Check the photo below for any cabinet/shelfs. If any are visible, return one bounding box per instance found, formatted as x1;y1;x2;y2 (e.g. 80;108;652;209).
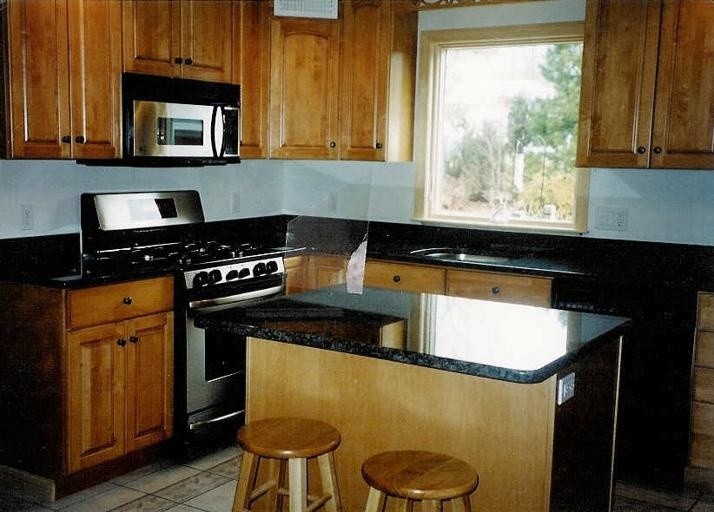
574;1;714;169
283;254;553;309
124;0;233;80
233;0;270;160
1;253;177;479
689;288;713;475
270;1;418;165
2;1;124;165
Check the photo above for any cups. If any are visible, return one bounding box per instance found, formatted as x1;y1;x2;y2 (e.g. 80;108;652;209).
543;205;556;221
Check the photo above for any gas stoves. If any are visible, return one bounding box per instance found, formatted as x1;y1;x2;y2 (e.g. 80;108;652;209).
84;239;285;290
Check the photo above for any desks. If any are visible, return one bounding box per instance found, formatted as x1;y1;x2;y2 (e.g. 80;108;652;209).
189;280;633;512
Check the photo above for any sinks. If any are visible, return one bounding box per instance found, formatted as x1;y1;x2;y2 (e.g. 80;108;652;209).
414;247;526;266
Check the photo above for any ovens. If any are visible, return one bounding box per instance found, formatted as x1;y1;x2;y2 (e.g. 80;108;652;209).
175;284;287;431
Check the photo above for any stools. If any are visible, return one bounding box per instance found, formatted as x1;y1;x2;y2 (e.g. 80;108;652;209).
359;446;482;511
226;412;350;511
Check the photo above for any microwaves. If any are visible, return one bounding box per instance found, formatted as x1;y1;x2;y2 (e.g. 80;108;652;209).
122;72;240;166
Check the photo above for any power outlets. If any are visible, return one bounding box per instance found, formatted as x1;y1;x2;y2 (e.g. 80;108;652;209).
556;371;576;406
